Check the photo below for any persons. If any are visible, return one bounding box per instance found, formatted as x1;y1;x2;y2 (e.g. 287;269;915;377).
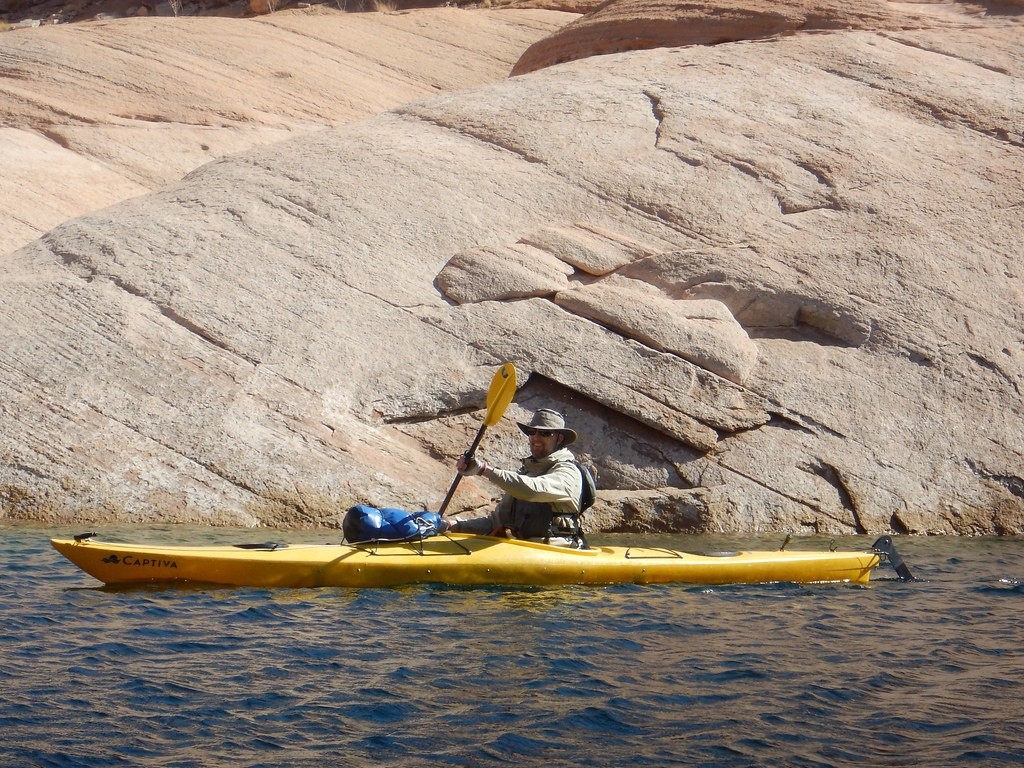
441;409;590;550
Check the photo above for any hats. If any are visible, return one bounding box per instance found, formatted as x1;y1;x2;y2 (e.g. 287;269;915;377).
515;408;577;447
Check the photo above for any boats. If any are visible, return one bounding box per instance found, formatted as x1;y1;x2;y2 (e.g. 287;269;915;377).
50;504;914;585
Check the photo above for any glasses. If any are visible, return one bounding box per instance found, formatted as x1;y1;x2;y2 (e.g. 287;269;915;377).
527;427;553;437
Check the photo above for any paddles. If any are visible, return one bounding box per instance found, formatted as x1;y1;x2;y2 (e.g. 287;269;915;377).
439;363;517;517
